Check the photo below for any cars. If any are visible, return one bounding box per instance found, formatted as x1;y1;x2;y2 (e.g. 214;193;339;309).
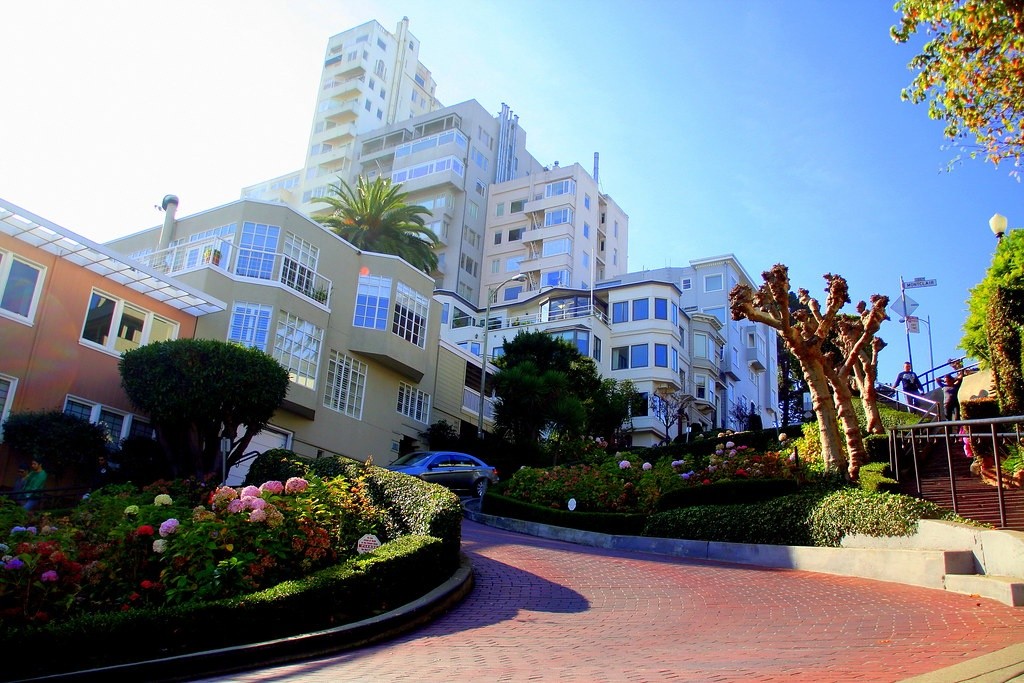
379;450;501;499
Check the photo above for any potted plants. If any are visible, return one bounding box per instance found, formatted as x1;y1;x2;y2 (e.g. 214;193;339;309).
313;284;327;304
205;248;221;266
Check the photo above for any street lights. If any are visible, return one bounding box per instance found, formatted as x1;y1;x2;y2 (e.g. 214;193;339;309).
476;273;529;440
898;314;936;390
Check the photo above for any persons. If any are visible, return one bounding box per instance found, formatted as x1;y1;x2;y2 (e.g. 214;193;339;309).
936;375;963;444
891;361;925;414
89;453;114;494
11;457;47;511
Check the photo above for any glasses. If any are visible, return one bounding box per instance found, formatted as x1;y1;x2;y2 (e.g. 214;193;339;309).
17;470;24;473
98;459;104;461
